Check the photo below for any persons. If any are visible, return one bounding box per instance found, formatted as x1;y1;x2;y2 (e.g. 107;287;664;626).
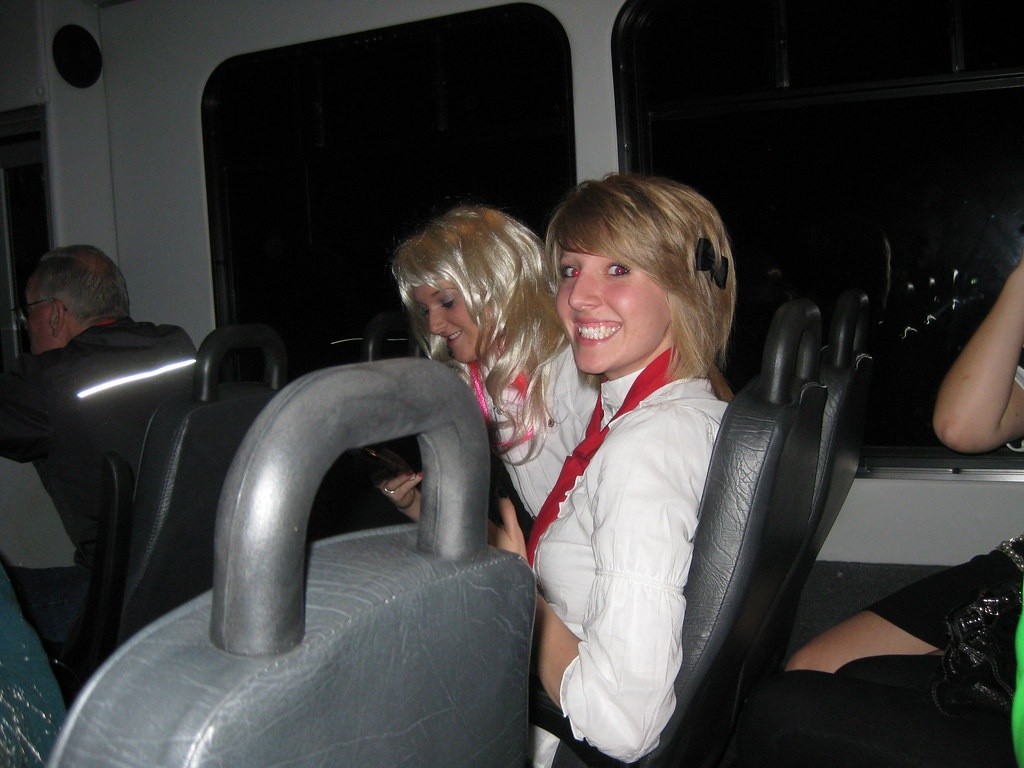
487;172;735;768
374;204;607;544
0;243;202;660
785;254;1024;674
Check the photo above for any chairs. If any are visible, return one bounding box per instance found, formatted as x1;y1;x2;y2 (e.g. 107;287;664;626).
47;287;873;768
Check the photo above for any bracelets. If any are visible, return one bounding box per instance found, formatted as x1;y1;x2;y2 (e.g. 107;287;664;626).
396;489;415;509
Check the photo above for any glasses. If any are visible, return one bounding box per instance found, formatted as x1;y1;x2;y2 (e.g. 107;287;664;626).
12;298;67;323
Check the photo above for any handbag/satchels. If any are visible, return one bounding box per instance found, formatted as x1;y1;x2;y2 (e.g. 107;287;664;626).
931;573;1022;720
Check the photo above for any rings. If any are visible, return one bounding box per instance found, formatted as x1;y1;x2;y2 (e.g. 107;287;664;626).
383;486;395;494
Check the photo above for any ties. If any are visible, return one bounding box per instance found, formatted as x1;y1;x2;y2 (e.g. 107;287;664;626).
526;345;676;566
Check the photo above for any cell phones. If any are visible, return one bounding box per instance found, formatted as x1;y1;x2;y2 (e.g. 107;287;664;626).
361;447;411;475
